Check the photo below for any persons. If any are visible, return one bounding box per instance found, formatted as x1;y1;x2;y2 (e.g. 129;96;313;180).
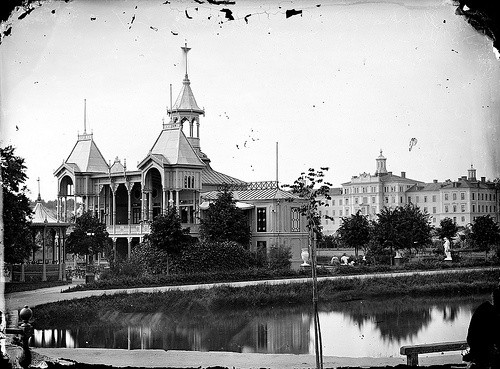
341;253;350;265
461;284;500;369
362;253;367;262
0;311;12;369
